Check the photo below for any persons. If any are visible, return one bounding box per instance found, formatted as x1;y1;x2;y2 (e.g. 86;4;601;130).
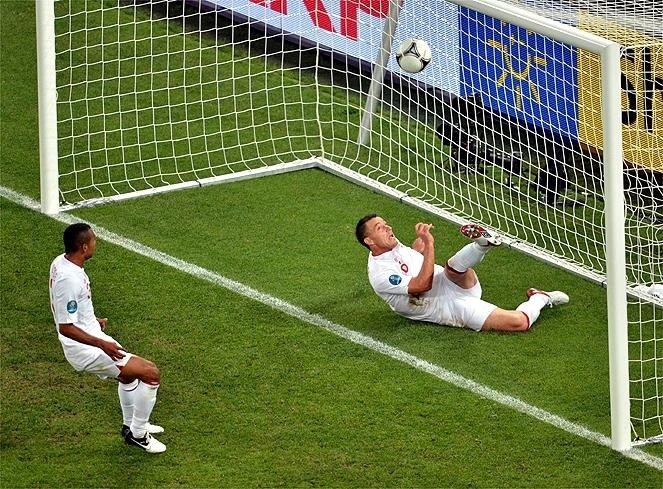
49;223;166;454
356;214;570;332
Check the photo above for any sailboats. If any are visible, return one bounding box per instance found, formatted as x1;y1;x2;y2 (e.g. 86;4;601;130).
396;39;431;73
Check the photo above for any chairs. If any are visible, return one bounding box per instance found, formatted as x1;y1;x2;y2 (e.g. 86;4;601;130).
125;431;168;454
121;423;165;438
526;286;571;308
459;223;501;248
